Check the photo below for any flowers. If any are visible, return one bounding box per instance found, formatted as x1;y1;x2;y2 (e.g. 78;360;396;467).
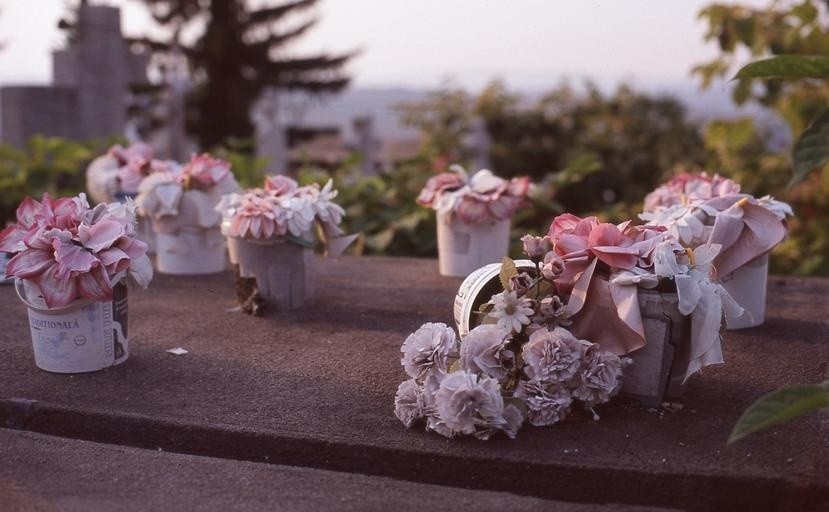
214;173;362;263
84;137;241;252
417;163;542;221
395;232;626;444
0;193;155;310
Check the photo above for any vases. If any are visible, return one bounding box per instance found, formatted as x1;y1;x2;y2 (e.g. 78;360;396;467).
590;273;685;411
233;234;319;310
153;227;229;276
451;256;542;341
11;277;133;376
